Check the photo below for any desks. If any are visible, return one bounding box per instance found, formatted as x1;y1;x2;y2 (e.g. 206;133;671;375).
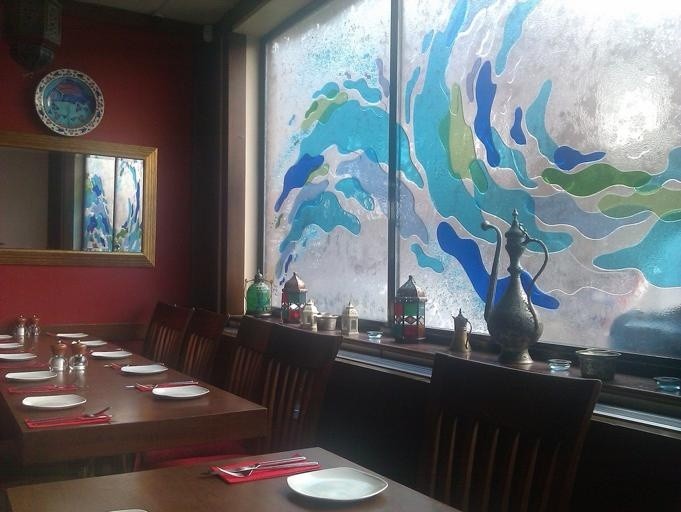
0;333;269;467
5;447;461;512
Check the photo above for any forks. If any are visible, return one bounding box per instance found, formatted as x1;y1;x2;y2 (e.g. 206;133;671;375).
217;456;307;477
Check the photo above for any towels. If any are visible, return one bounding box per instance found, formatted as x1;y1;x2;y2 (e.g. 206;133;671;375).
0;333;200;429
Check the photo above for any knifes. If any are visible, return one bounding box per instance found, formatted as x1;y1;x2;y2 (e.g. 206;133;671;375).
33;414;113;425
210;462;319;475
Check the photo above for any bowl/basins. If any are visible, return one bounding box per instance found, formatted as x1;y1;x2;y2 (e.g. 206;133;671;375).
654;376;681;390
368;331;383;338
548;358;572;371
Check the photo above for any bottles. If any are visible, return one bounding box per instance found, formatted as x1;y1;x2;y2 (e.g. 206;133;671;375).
48;339;69;371
65;338;88;370
29;313;42;335
15;314;29;335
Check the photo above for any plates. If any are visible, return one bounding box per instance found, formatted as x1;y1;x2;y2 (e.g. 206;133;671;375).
57;333;89;338
33;67;103;138
90;350;133;359
121;364;169;373
5;371;58;381
1;335;12;339
0;353;37;361
23;393;87;409
1;343;20;349
287;466;390;502
72;340;107;346
153;386;210;398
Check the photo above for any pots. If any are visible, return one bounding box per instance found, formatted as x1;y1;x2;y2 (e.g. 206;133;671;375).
314;313;339;332
575;347;622;381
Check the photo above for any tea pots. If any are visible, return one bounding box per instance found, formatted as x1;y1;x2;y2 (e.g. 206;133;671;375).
482;210;551;367
450;308;474;353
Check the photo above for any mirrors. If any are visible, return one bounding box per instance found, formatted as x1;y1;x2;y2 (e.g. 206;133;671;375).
0;131;159;268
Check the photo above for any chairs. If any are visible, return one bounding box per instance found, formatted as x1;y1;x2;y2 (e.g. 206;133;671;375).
136;299;345;452
416;351;603;512
